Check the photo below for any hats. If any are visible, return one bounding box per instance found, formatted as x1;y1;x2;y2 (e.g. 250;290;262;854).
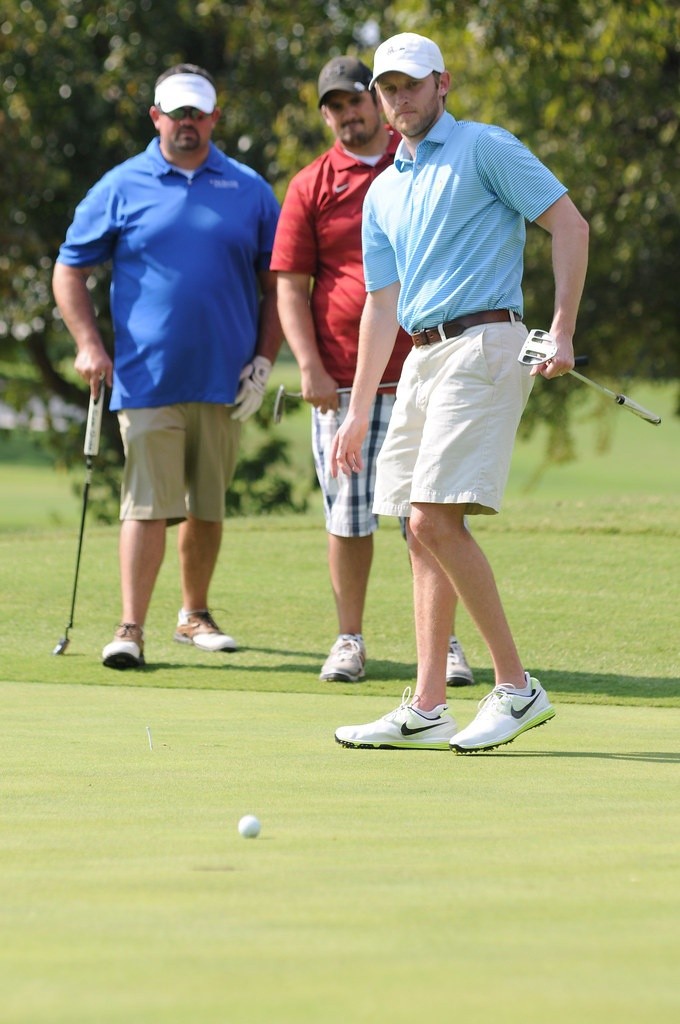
317;56;373;109
367;32;445;92
153;63;218;114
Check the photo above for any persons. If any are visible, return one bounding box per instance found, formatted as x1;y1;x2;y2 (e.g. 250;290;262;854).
331;32;589;753
270;56;474;686
52;62;286;671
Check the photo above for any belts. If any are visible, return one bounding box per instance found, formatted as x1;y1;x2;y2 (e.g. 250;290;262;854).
411;309;523;348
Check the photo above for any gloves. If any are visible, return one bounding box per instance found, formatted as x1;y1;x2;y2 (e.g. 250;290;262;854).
223;355;273;425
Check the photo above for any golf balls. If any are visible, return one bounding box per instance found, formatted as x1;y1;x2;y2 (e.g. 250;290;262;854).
237;815;262;839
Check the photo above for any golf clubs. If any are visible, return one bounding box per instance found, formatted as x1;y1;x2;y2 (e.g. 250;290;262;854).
272;380;399;427
54;377;107;655
517;328;663;430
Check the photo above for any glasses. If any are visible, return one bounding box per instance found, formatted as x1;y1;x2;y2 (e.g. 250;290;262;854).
157;105;208;122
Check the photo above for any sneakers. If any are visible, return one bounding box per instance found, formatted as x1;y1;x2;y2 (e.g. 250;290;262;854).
100;623;146;668
170;607;237;653
319;634;369;682
334;686;457;752
446;633;475;688
449;671;556;756
